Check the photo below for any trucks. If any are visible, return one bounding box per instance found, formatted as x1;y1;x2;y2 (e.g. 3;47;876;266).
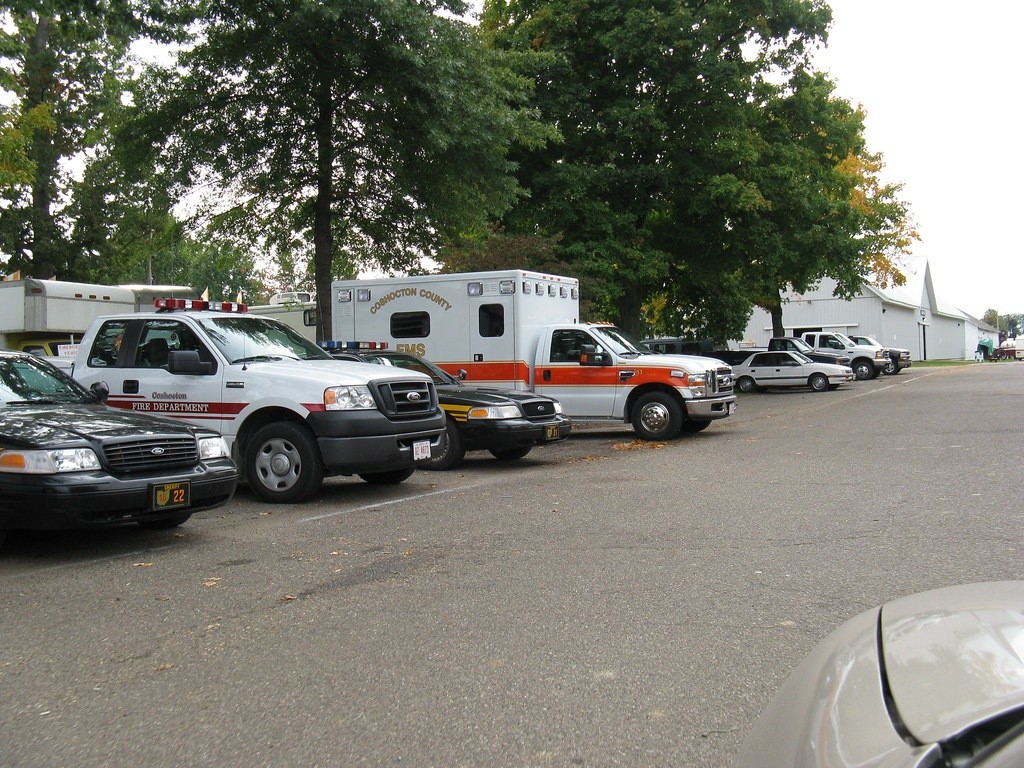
327;267;739;441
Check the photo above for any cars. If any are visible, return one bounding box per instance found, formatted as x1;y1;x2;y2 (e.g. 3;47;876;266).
730;351;854;393
832;334;913;375
316;340;573;473
0;348;239;536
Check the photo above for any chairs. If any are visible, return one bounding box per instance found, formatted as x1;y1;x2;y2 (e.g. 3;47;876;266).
142;337;170;368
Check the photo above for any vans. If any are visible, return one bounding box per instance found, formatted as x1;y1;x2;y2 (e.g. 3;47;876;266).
0;276;202;363
639;337;729;359
242;291;318;344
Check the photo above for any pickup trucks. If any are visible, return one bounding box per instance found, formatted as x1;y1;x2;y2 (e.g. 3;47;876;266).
739;330;892;382
33;298;448;504
700;336;850;368
